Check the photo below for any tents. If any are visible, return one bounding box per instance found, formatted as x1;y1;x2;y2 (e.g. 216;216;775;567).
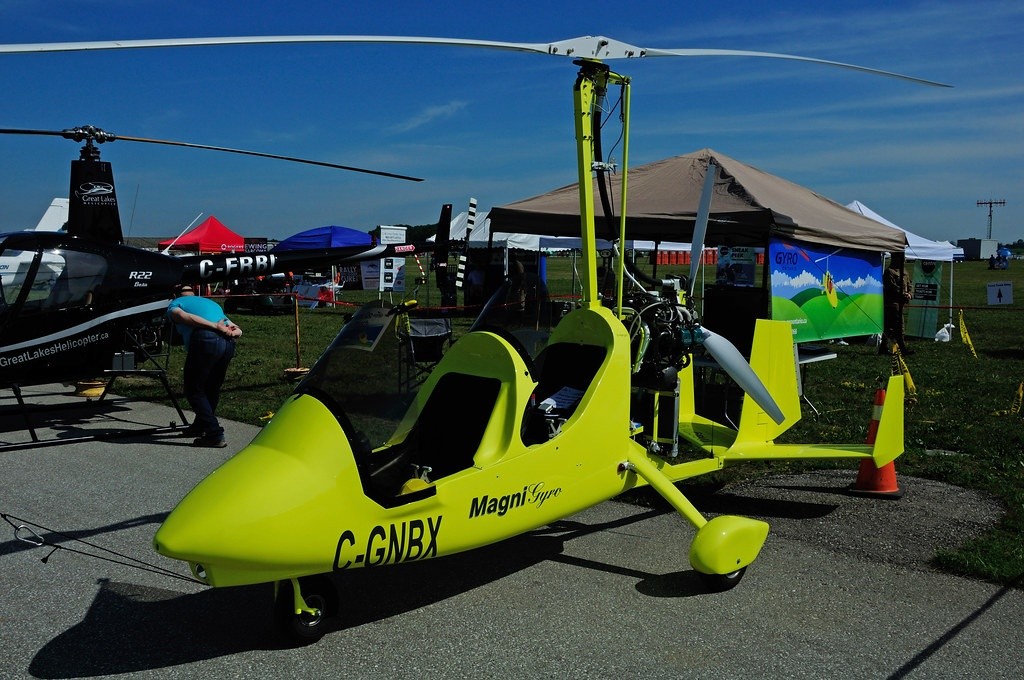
425;149;964;340
155;204;253;282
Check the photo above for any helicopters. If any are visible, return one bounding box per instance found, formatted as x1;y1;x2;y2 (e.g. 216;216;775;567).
0;32;957;627
1;125;454;390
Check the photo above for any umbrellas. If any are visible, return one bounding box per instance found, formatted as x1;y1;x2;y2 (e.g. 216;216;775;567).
269;225;381;308
997;248;1012;256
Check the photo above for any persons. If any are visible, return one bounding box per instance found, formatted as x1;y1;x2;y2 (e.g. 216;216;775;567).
989;255;996;269
166;284;243;448
996;255;1006;270
226;269;277;295
878;252;916;357
334;268;341;293
284;271;293;288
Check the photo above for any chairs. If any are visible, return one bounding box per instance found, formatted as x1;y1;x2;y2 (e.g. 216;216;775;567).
397;316;455;394
374;372;502;496
45;266;95;313
533;342;609;444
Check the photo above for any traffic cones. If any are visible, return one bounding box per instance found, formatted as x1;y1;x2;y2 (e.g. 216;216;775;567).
849;389;907;501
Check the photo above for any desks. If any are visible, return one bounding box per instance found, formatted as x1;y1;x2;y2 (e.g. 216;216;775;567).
693;351;839;431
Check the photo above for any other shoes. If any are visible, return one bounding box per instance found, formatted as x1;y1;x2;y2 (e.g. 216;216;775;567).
877;349;894;356
193;434;227;448
901;349;916;355
182;425;205;436
828;339;834;345
836;341;849;346
